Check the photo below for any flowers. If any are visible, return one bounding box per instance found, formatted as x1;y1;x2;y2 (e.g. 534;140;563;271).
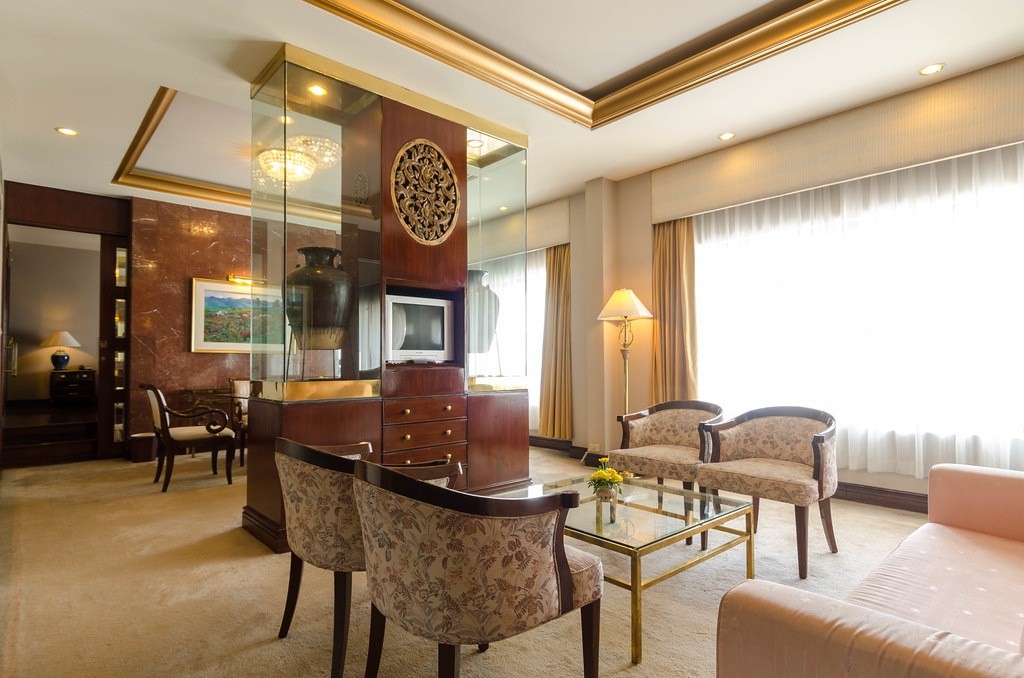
586;457;623;495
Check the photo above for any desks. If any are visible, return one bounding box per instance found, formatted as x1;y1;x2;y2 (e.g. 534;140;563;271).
490;474;754;664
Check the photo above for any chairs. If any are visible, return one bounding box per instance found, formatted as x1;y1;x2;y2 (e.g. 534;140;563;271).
352;458;605;678
700;406;838;579
228;378;251;466
275;435;463;678
610;400;723;545
138;382;236;493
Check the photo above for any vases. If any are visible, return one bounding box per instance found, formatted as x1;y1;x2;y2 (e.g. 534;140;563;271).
596;486;616;502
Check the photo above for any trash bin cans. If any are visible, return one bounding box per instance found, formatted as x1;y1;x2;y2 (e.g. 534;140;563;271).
127;433;157;463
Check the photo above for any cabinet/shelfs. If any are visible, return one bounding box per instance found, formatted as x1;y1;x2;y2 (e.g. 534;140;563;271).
241;389;534;552
49;370;97;405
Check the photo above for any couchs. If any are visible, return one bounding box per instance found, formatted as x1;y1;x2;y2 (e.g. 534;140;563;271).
715;462;1024;678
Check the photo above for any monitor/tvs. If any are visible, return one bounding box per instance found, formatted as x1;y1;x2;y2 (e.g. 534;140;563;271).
385;294;454;365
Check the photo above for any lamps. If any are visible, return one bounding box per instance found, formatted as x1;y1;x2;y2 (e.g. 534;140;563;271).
40;331;81;371
597;288;653;479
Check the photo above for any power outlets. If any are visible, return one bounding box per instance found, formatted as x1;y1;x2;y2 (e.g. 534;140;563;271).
590;444;600;452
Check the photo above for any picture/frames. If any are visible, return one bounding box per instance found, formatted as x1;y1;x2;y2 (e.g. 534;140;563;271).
191;277;296;355
